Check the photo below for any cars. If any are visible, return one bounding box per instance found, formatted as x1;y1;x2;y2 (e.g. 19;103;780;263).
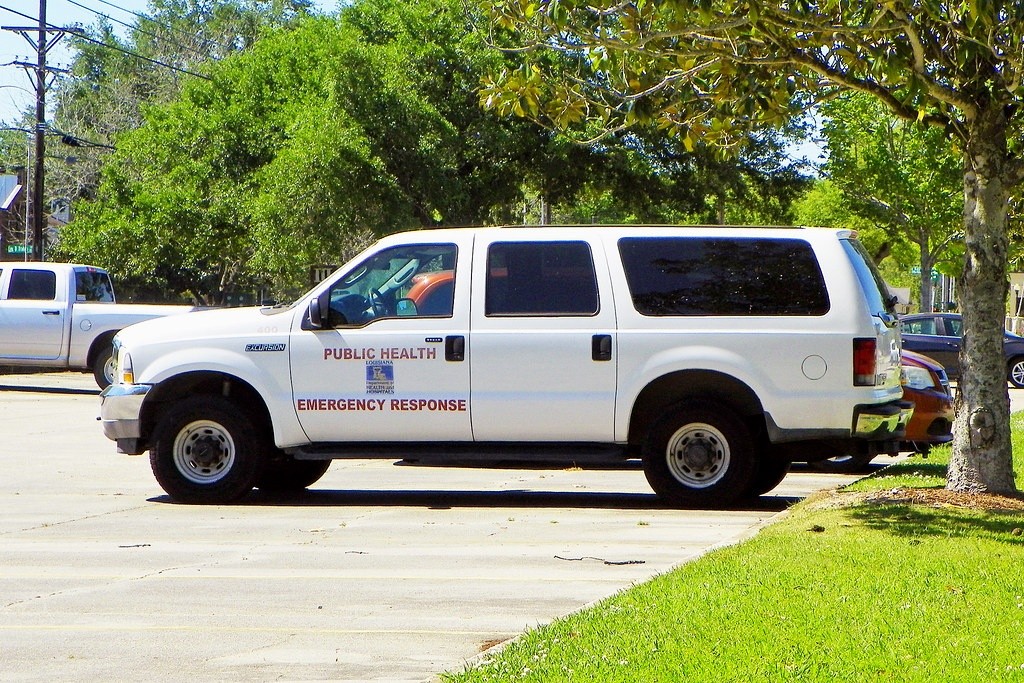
932;301;955;312
396;263;956;460
895;312;1024;387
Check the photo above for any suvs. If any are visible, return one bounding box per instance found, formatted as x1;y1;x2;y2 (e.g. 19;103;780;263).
101;222;916;512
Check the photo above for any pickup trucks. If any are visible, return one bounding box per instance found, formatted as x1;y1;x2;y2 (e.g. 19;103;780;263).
0;260;223;394
